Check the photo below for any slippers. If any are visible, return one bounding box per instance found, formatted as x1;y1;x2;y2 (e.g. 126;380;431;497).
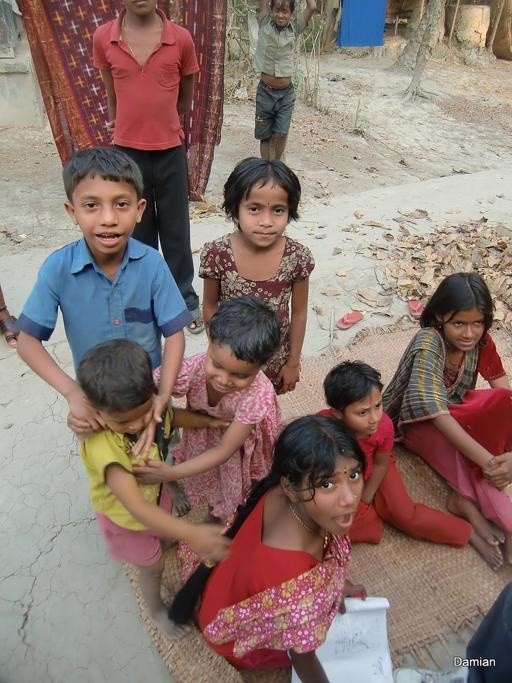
409;300;423;316
337;310;363;329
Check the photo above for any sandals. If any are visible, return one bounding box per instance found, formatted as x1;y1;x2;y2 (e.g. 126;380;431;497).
185;307;205;333
1;305;20;342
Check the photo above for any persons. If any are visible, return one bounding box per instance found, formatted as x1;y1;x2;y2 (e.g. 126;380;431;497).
252;0;317;161
0;285;17;348
17;147;512;683
92;0;204;334
